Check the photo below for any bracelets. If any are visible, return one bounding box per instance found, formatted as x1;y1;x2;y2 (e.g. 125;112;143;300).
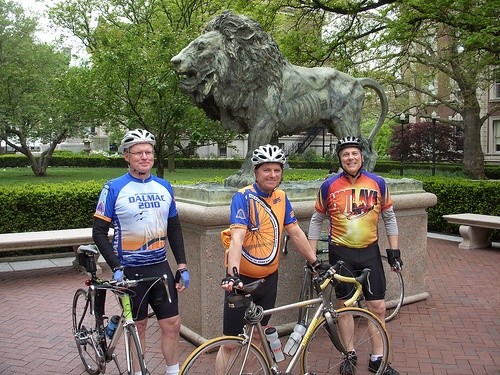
311;260;320;266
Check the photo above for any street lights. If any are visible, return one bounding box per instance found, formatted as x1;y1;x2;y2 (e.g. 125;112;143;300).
398;113;405;176
431;111;437;176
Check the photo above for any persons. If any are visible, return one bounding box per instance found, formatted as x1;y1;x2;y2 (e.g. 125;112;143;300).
215;144;336;375
308;136;403;375
92;128;190;375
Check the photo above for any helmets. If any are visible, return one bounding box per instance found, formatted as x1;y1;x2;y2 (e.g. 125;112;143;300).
250;144;287;166
120;127;156;155
335;135;364;153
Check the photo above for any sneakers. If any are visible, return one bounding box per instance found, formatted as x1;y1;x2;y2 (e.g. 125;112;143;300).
339;351;358;375
368;353;400;375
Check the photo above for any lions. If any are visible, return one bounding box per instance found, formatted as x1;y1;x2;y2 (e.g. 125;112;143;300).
170;9;389;188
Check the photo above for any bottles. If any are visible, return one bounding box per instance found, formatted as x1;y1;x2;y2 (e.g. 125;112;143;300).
283;320;307;356
264;325;285;363
105;315;120;339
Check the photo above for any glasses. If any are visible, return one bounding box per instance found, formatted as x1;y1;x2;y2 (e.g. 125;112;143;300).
128;150;156;158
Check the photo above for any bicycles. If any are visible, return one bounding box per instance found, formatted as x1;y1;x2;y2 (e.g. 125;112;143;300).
177;261;392;375
281;232;405;328
70;243;172;375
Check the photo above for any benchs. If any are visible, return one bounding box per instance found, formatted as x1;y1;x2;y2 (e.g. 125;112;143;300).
0;227;116;276
443;214;500;250
